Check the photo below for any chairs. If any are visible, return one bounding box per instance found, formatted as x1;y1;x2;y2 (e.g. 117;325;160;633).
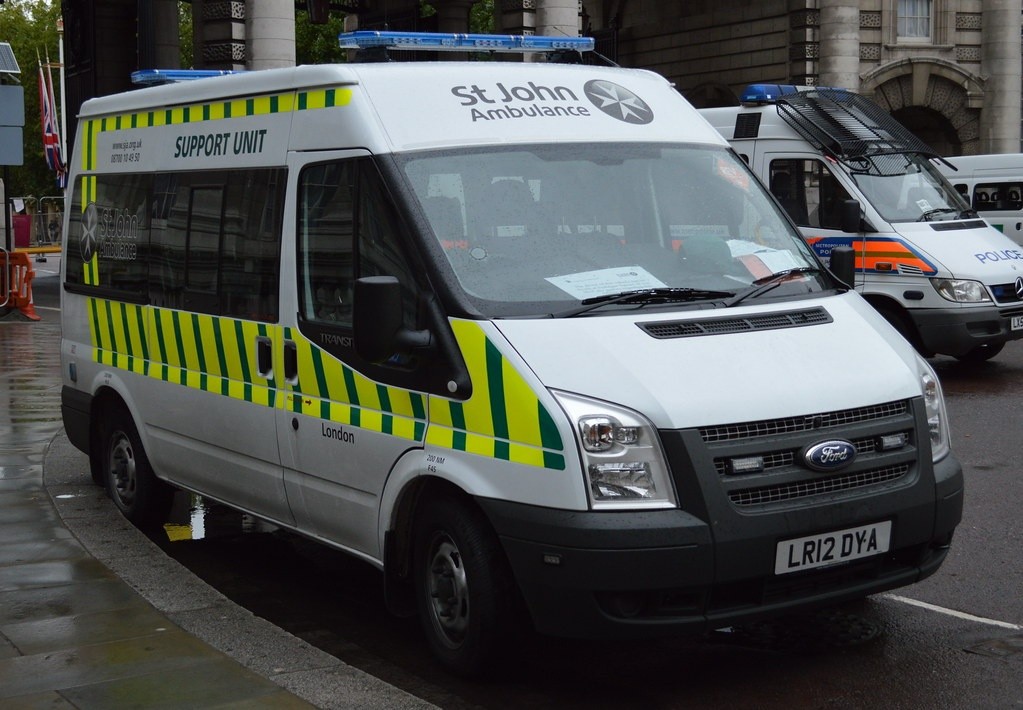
769;170;807;227
472;179;537;258
420;193;466;242
822;175;852;228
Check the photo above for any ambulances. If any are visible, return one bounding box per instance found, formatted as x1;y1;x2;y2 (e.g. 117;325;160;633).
58;30;964;691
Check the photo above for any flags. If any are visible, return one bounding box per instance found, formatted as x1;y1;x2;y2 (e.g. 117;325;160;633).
39;59;69;188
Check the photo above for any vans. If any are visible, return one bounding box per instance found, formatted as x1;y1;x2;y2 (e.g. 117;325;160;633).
927;153;1023;246
498;81;1022;359
